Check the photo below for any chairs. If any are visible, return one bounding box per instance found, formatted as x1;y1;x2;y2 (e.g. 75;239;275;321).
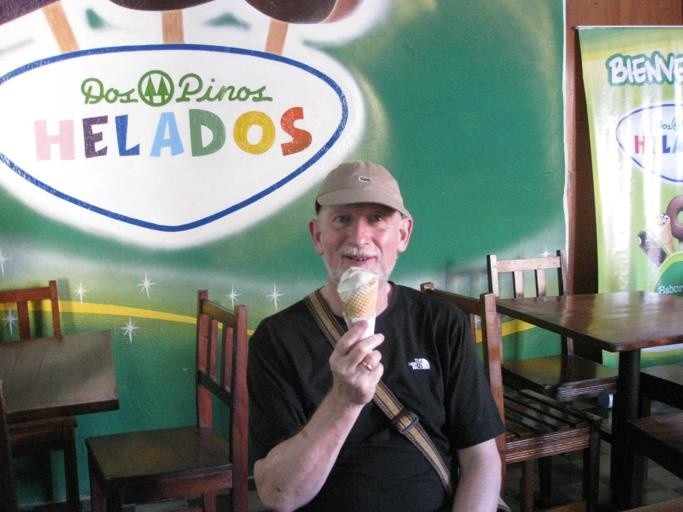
84;290;249;511
486;248;618;411
416;281;602;511
0;280;79;511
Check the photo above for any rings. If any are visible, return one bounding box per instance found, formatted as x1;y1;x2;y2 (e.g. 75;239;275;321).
361;361;374;373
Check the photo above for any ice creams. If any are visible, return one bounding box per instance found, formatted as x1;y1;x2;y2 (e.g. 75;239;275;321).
337;266;378;339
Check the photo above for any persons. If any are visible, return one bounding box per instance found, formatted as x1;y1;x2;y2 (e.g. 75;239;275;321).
244;158;507;512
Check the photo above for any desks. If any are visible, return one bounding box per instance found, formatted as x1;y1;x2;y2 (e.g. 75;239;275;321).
494;291;682;510
0;329;118;511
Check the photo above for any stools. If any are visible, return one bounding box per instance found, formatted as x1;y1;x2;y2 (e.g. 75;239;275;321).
625;411;682;511
638;361;682;479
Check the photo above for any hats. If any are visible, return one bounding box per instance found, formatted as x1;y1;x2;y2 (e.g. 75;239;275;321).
317;159;410;218
